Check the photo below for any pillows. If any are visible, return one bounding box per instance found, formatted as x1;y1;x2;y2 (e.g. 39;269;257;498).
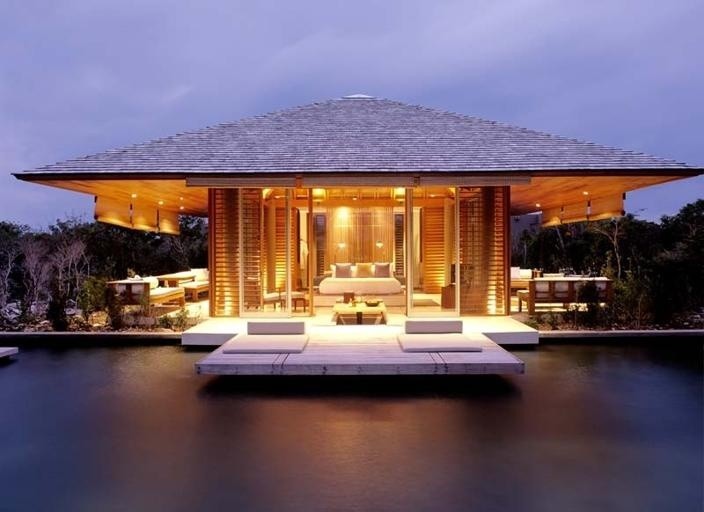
329;262;394;277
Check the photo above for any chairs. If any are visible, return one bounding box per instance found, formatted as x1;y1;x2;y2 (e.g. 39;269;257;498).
244;279;306;313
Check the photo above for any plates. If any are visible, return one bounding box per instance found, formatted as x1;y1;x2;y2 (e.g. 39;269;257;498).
364;301;379;307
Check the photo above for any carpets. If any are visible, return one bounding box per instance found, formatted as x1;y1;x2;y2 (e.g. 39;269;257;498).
279;299;309;307
412;298;440;306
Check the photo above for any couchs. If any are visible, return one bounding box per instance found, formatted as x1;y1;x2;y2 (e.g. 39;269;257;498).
509;266;614;315
106;269;210;317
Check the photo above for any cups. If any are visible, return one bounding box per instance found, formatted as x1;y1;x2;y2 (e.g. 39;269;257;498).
352;301;356;306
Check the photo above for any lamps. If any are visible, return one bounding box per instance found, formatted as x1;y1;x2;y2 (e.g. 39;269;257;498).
311;188;327;203
393;187;406;204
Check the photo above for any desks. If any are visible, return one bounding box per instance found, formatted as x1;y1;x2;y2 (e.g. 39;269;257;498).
331;301;389;325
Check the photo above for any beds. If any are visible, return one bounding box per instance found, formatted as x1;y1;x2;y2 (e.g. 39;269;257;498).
318;277;404;294
